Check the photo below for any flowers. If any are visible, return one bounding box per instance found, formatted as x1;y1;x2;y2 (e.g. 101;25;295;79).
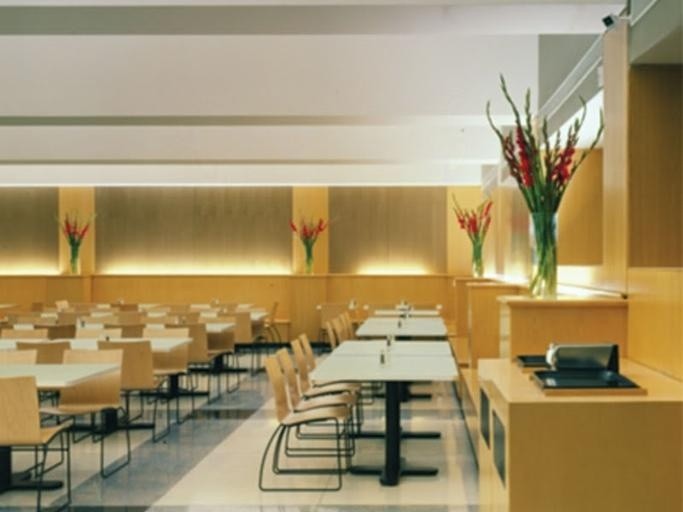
50;209;102;273
286;212;330;269
485;72;595;295
449;192;493;273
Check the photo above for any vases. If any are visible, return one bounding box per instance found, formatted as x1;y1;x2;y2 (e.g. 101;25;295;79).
69;243;79;276
528;211;556;298
470;236;481;277
304;245;313;274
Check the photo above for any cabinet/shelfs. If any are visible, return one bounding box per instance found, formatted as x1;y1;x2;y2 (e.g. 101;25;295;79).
477;354;683;512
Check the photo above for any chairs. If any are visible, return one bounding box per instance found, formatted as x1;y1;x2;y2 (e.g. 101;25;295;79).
130;297;253;443
0;298;130;512
253;299;384;492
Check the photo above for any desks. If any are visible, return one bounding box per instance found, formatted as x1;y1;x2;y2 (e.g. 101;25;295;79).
308;309;460;487
